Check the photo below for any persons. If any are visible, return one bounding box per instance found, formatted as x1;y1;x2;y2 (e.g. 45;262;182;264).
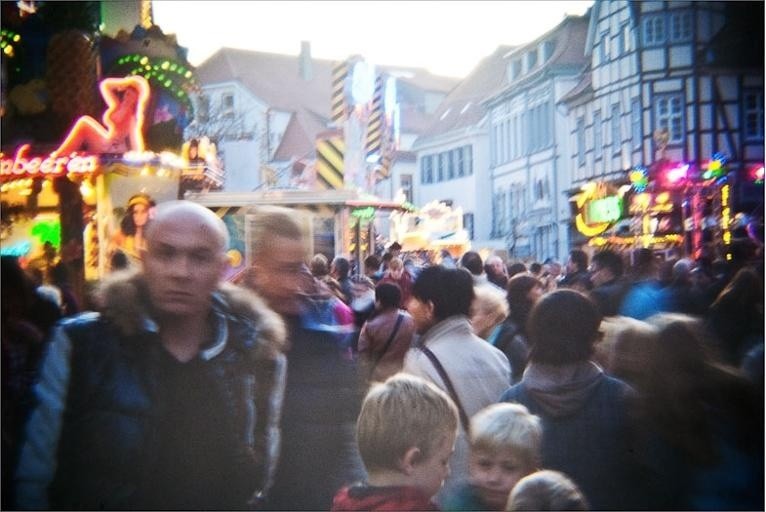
54;74;151;156
1;200;765;511
103;191;155;275
188;138;205;164
